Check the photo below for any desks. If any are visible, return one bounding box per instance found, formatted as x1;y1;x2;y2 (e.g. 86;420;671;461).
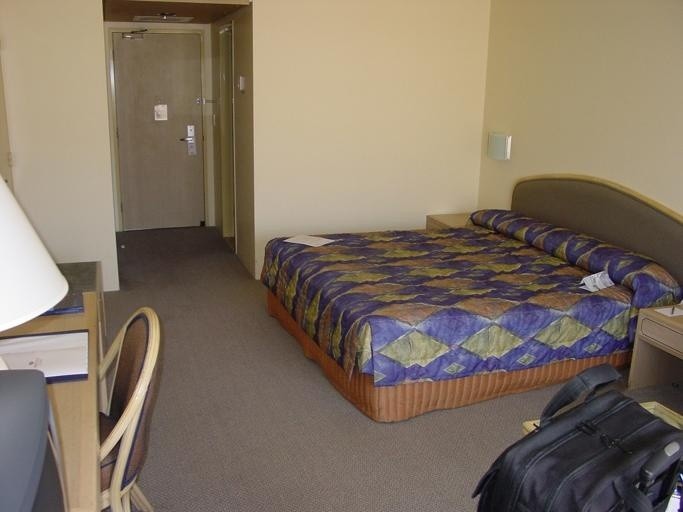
0;261;107;512
522;401;683;434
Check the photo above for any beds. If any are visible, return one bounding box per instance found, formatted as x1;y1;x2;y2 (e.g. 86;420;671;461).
261;173;683;424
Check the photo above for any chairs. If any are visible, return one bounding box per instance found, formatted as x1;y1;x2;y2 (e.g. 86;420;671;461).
100;307;163;511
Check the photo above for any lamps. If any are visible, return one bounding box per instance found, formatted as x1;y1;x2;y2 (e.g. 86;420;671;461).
0;175;69;369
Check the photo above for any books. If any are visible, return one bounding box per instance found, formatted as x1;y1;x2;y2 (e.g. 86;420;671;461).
40;291;84;316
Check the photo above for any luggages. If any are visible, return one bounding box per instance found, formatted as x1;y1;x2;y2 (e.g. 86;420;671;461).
470;362;683;511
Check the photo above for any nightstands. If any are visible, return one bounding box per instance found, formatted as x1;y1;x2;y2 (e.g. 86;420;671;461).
627;304;682;393
426;213;471;229
